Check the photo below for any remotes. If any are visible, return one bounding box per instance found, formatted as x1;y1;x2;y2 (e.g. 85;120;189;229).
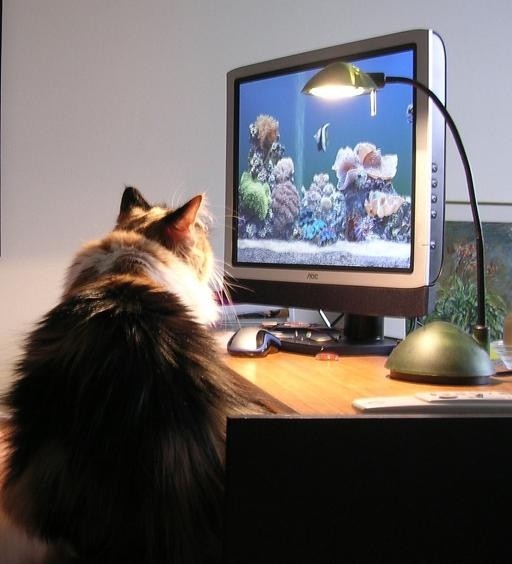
352;391;512;414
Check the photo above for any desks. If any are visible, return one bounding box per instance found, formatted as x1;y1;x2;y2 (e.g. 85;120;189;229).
202;324;511;414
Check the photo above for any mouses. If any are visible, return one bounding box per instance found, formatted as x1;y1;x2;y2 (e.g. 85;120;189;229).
227;324;282;358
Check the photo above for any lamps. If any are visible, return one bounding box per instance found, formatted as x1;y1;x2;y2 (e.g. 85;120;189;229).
301;61;499;384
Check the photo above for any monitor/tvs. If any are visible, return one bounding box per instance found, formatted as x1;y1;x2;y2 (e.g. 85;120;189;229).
223;29;448;356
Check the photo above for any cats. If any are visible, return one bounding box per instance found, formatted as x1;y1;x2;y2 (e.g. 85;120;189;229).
1;183;262;564
